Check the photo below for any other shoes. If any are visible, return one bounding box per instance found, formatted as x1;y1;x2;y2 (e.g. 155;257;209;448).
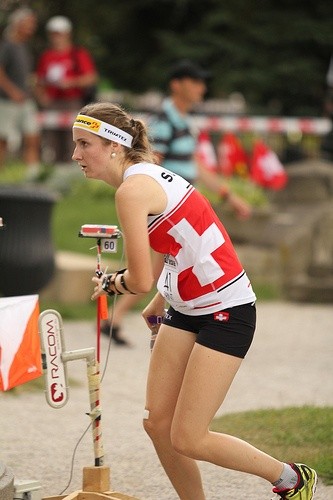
100;319;126;345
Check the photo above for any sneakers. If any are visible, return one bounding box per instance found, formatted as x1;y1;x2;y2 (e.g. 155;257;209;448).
272;462;318;500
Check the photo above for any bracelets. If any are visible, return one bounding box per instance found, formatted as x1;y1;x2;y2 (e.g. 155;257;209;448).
109;273;124;295
120;272;136;295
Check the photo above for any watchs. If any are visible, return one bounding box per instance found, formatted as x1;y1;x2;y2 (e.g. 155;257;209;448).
102;275;115;297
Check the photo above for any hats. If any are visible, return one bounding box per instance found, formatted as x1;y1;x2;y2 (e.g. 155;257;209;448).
171;60;212;79
46;15;72;32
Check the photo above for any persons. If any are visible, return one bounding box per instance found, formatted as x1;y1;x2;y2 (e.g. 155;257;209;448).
1;7;100;184
70;101;319;500
98;68;252;346
